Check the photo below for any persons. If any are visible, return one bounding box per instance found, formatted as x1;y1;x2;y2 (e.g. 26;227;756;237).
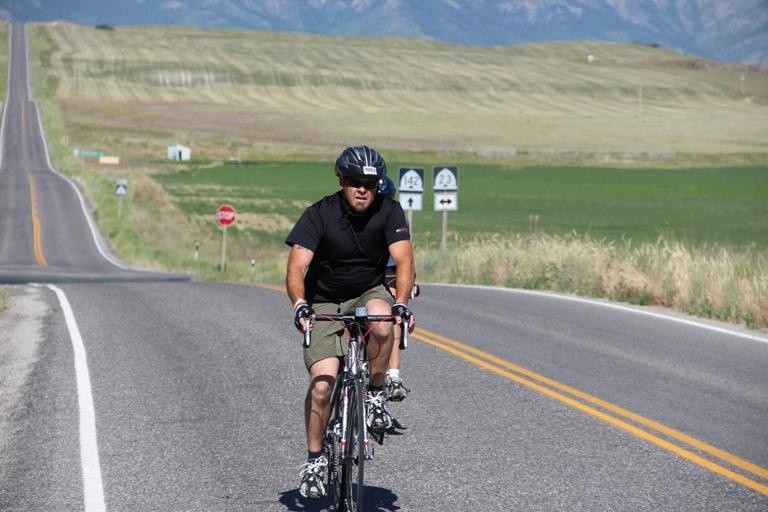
284;146;418;499
377;175;406;401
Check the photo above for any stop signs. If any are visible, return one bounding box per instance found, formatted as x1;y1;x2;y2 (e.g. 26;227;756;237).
216;206;238;227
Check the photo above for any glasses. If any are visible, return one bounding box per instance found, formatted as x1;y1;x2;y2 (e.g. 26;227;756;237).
349;179;377;189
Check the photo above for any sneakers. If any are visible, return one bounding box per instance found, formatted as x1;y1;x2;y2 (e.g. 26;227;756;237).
365;377;408;432
299;455;329;499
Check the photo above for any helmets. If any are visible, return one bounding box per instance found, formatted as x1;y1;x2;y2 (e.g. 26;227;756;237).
376;176;396;195
335;145;386;177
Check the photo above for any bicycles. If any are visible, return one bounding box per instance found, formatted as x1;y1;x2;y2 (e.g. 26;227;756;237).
294;284;419;510
